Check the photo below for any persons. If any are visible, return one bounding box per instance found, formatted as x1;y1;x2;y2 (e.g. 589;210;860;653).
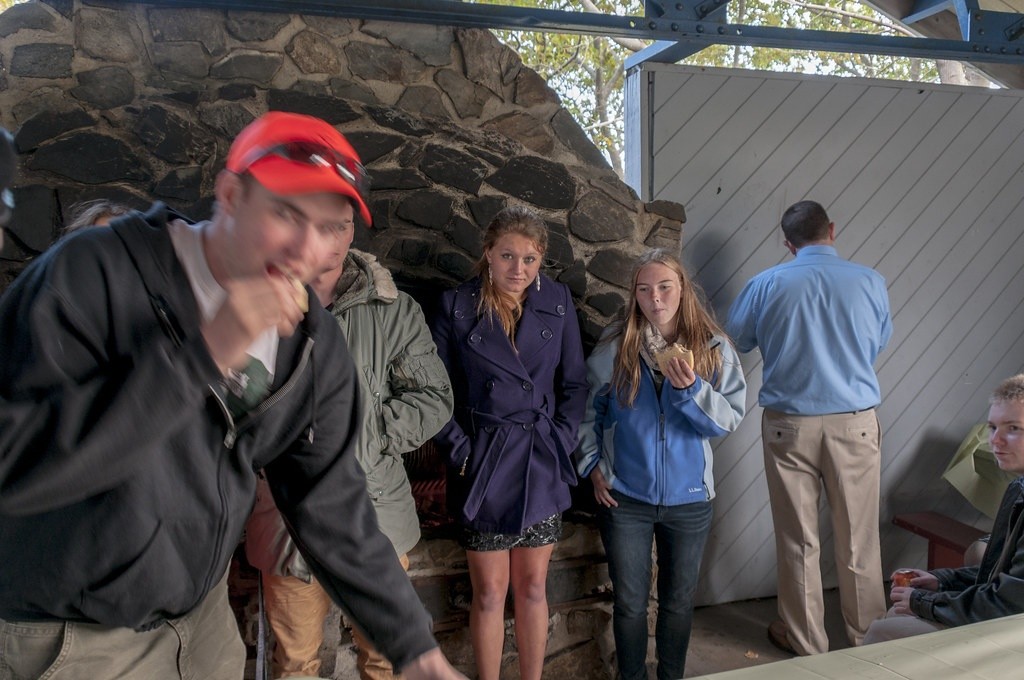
432;206;588;680
859;371;1024;647
727;201;891;655
574;250;746;680
0;111;472;680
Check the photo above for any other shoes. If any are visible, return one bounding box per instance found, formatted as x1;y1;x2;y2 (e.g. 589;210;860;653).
768;621;799;655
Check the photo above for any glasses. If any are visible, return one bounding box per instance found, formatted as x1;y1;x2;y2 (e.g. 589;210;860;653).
236;141;375;201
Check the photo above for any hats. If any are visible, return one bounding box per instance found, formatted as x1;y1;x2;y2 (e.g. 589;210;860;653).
226;110;376;229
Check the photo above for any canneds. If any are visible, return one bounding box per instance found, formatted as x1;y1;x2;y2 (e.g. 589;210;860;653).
894;570;918;588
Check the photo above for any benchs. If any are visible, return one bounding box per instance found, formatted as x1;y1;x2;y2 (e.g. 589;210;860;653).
891;511;992;570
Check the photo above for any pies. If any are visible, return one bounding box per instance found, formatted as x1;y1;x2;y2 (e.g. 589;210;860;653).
654;342;694;376
